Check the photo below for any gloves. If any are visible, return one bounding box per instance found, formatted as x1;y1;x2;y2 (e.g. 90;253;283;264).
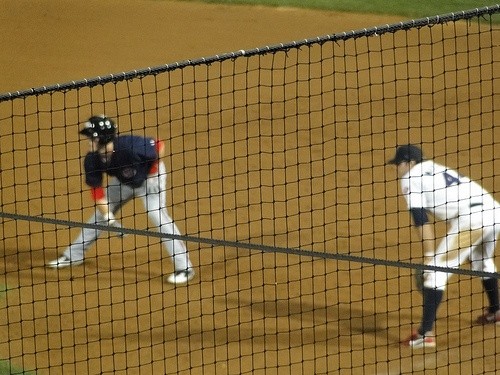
104;211;128;237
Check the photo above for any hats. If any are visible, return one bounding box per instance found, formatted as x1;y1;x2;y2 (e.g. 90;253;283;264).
387;145;423;166
79;115;116;144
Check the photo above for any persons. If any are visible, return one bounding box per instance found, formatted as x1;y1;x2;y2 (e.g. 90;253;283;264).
387;145;500;349
43;114;195;284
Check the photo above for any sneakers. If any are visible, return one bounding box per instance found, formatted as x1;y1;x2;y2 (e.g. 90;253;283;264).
404;330;436;350
477;308;499;325
166;268;194;285
46;254;82;268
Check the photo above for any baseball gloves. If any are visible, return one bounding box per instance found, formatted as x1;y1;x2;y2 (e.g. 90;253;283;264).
415;263;424;294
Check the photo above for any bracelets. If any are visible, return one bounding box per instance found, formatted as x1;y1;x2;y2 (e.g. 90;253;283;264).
103;212;115;226
424;252;435;257
